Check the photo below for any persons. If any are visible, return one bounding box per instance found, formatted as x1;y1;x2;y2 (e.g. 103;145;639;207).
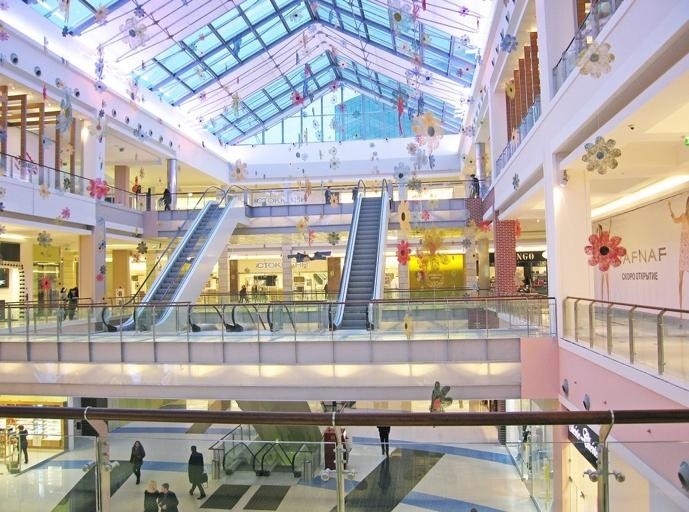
470;174;479;198
352;187;357;202
160;189;171;211
377;428;390;458
15;424;28;463
324;283;328;299
325;187;333;204
143;481;160;512
239;285;248;303
130;440;145;485
157;482;179;512
188;446;206;500
136;185;141;194
58;287;78;320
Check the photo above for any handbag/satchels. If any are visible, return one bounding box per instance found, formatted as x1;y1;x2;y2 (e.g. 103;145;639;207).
199;473;208;483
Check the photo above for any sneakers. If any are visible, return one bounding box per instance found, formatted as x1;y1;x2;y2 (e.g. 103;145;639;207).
190;491;193;495
197;495;205;499
